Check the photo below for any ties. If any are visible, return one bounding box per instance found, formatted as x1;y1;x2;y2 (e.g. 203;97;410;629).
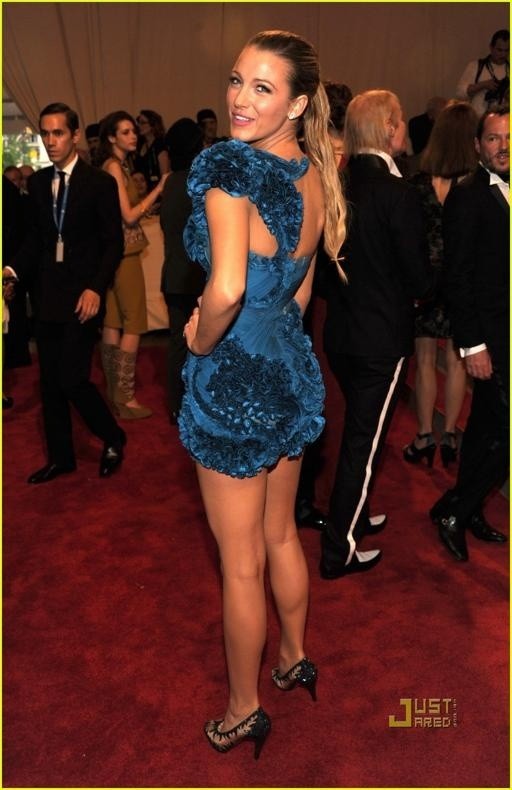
53;171;67;228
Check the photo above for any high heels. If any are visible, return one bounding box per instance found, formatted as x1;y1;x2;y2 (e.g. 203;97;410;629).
272;657;319;702
438;432;458;467
402;433;436;469
205;705;271;761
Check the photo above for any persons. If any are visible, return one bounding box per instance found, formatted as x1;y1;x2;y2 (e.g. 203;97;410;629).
425;101;510;566
174;23;355;758
314;84;445;582
452;27;511;98
3;78;510;534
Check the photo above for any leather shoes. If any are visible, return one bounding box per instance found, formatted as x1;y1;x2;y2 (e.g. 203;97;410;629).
27;462;76;484
430;507;469;561
300;510;328;528
469;517;508;543
367;515;388;534
99;428;127;478
321;549;381;579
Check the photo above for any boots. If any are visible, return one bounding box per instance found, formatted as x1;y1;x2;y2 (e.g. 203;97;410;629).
100;344;152;419
